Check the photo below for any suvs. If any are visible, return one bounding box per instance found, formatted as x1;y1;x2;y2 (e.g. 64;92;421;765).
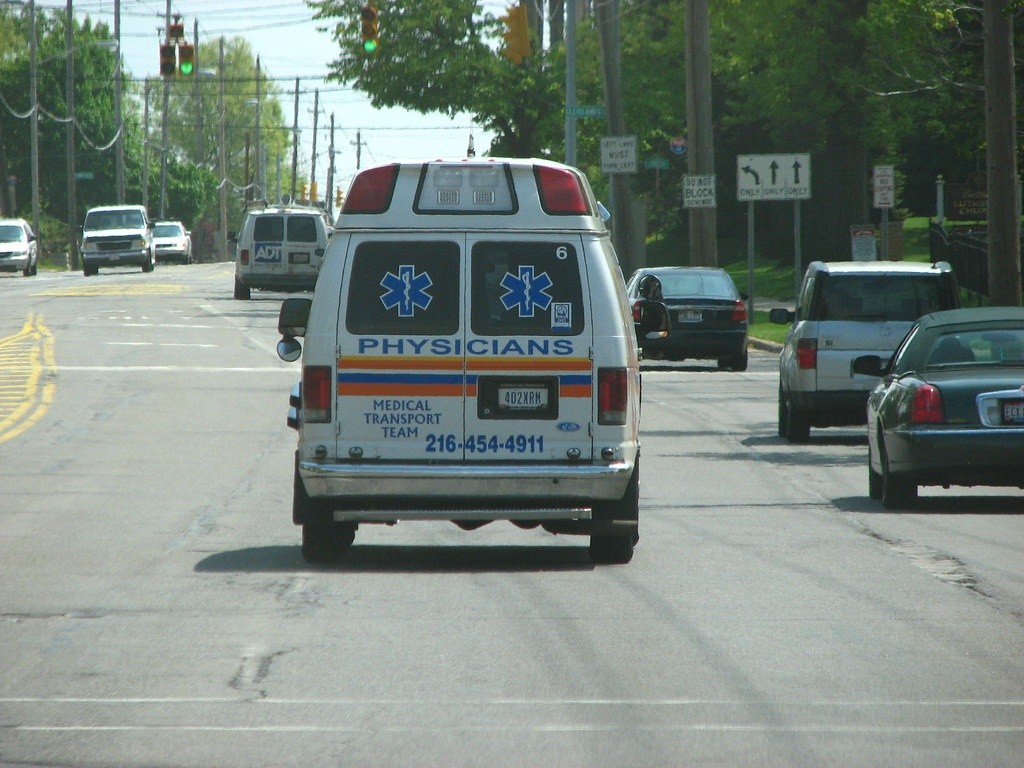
770;260;961;442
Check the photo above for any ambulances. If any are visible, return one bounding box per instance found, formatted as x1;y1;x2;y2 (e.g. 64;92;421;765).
272;155;674;572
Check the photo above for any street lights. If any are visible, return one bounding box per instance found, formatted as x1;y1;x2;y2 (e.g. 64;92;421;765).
142;68;217;210
28;37;119;275
197;98;258;267
244;126;301;220
289;149;344;203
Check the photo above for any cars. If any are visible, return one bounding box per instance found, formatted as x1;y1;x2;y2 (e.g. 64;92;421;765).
149;219;193;267
623;265;749;374
0;218;38;276
850;305;1024;508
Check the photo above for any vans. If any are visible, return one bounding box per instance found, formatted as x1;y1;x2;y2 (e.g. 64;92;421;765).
225;197;336;300
75;204;157;274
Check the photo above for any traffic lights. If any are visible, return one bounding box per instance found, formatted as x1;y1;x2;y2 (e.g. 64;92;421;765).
498;4;531;64
336;188;343;206
165;23;186;41
158;43;176;77
358;6;378;57
178;46;193;74
